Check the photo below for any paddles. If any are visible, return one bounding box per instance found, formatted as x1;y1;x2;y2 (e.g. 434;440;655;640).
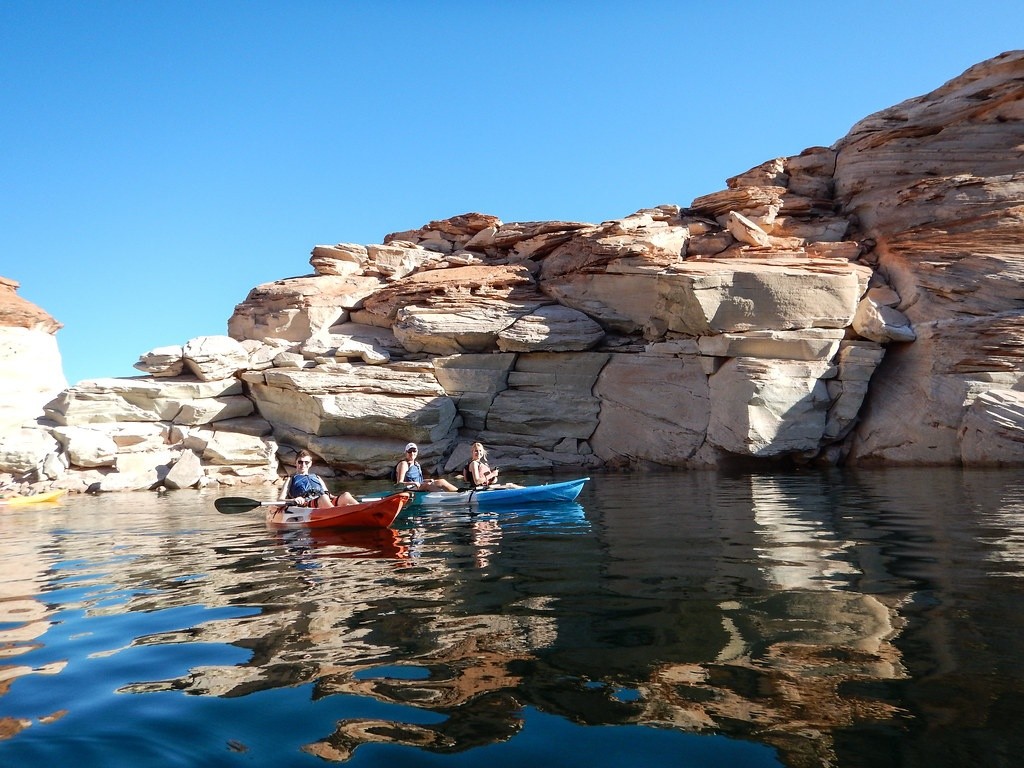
215;496;385;516
491;466;499;481
386;485;414;497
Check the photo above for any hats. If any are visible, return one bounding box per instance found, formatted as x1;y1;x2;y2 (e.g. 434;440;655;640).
405;443;417;451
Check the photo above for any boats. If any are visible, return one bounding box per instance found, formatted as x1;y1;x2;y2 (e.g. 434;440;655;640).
360;476;592;504
264;490;411;533
0;488;69;505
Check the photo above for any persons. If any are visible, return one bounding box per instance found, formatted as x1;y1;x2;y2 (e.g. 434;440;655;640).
465;442;525;490
278;449;360;508
391;443;459;492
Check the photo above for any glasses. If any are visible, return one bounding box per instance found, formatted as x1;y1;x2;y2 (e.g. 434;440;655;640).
407;449;416;453
298;461;310;464
